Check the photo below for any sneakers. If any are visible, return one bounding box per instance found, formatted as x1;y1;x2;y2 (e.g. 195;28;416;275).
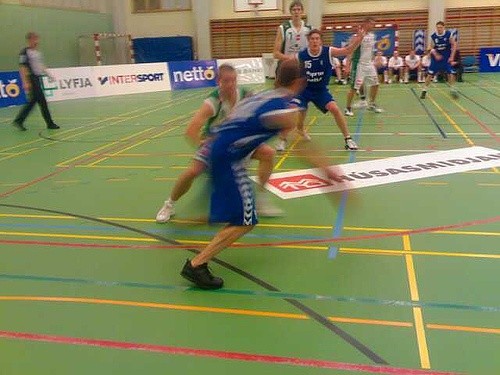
180;258;224;289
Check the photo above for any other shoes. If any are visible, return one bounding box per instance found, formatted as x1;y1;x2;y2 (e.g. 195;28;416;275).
256;201;284;218
295;129;311;141
156;200;175;223
366;104;382;112
344;137;358;150
420;91;427;99
344;107;353;116
14;119;26;131
47;123;59;129
275;141;285;150
450;90;459;98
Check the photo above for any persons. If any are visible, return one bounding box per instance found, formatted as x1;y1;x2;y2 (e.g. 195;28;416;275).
276;26;366;151
331;50;463;85
180;58;306;290
156;64;282;223
13;32;59;131
344;17;382;116
420;21;457;99
273;2;311;142
